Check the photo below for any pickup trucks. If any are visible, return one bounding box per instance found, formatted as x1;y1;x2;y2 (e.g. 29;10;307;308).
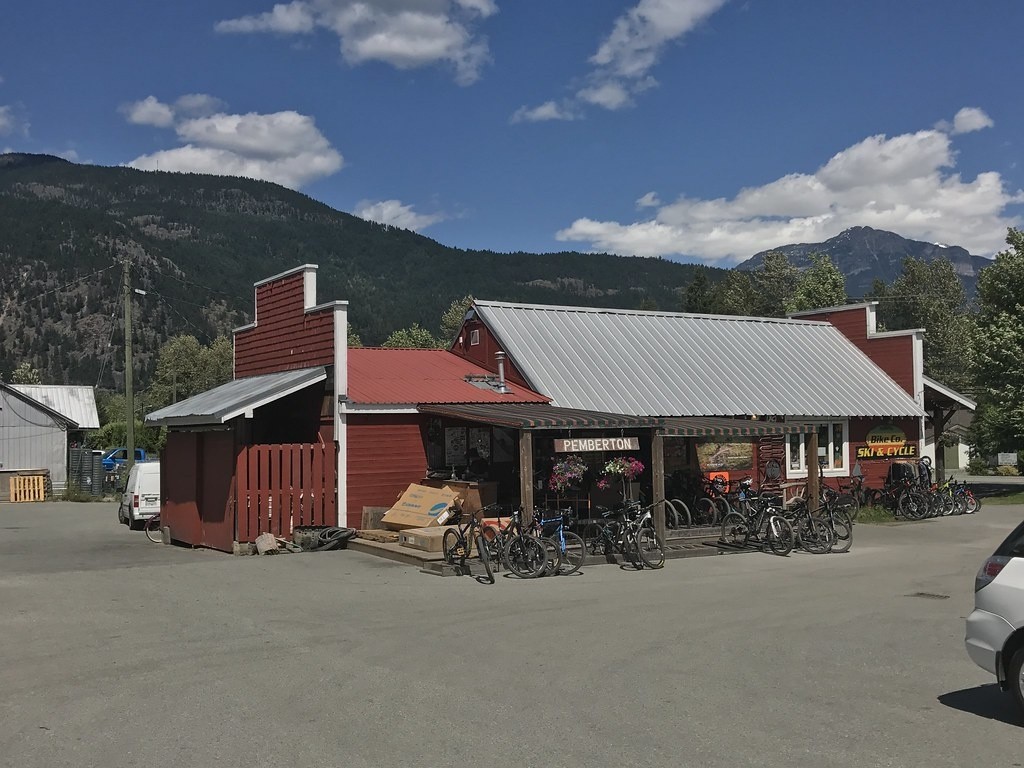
101;447;159;471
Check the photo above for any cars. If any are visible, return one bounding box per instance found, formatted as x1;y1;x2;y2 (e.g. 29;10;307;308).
962;518;1024;711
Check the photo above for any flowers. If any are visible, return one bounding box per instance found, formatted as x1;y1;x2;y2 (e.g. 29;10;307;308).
595;456;645;495
547;454;588;501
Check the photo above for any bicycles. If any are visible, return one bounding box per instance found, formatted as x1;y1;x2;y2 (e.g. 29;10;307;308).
492;455;983;578
142;513;162;543
441;502;503;584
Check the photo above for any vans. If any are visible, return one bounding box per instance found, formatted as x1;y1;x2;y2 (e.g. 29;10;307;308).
117;460;160;529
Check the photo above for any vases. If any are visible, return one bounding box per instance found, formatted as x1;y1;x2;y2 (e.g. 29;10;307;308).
569;478;582;491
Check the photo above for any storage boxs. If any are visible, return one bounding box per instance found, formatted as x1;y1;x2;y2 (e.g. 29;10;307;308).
380;482;536;553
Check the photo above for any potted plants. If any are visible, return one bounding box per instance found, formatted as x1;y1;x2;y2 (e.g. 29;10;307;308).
939;429;960;449
